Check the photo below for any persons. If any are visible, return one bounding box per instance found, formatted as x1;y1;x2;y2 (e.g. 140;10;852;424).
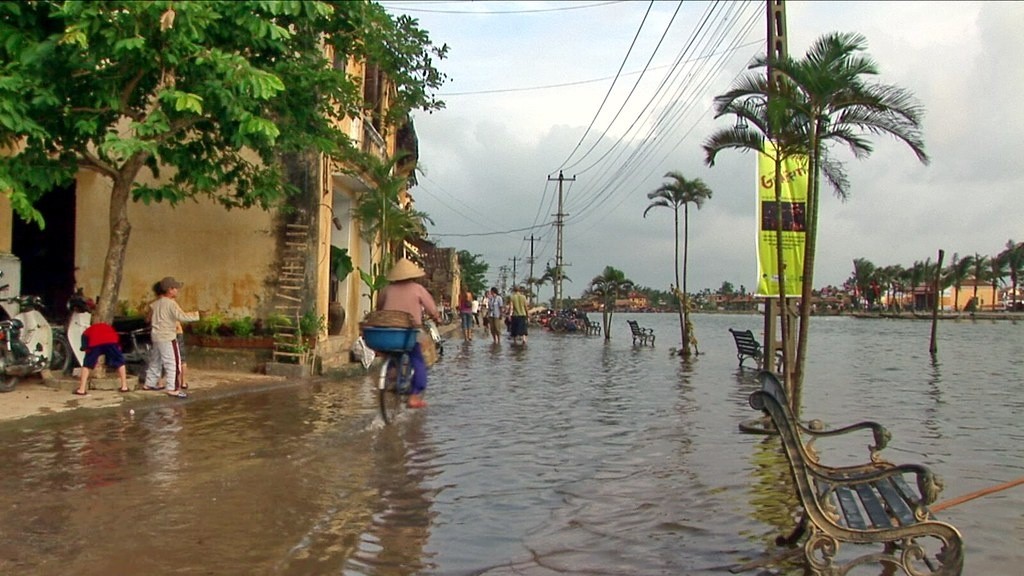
472;287;502;346
503;287;530;346
376;257;443;408
72;323;129;395
457;282;473;342
142;276;207;398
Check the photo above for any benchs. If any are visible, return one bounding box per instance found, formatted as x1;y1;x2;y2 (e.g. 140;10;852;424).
748;371;965;576
582;314;601;336
626;319;655;348
728;327;782;372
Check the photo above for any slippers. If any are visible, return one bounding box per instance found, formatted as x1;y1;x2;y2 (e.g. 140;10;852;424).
75;387;86;395
143;385;153;390
120;387;129;392
406;398;428;408
154;385;165;390
181;383;187;388
169;391;187;398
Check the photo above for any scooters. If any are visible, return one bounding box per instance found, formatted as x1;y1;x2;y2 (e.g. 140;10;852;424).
0;272;157;392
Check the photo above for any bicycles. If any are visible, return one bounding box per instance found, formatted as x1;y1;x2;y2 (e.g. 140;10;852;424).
377;315;432;423
532;307;596;332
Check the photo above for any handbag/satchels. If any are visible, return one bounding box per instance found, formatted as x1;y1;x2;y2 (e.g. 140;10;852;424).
465;301;472;308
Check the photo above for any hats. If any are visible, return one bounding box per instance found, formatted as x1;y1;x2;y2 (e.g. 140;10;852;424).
386;258;427;279
158;277;184;292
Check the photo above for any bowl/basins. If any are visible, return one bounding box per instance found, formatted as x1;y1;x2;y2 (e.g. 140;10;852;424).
363;328;421;352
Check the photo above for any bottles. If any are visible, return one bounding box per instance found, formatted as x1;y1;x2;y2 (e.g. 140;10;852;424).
328;301;345;335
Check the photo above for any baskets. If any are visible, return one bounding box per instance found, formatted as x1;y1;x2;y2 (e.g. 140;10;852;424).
364;310;427;328
